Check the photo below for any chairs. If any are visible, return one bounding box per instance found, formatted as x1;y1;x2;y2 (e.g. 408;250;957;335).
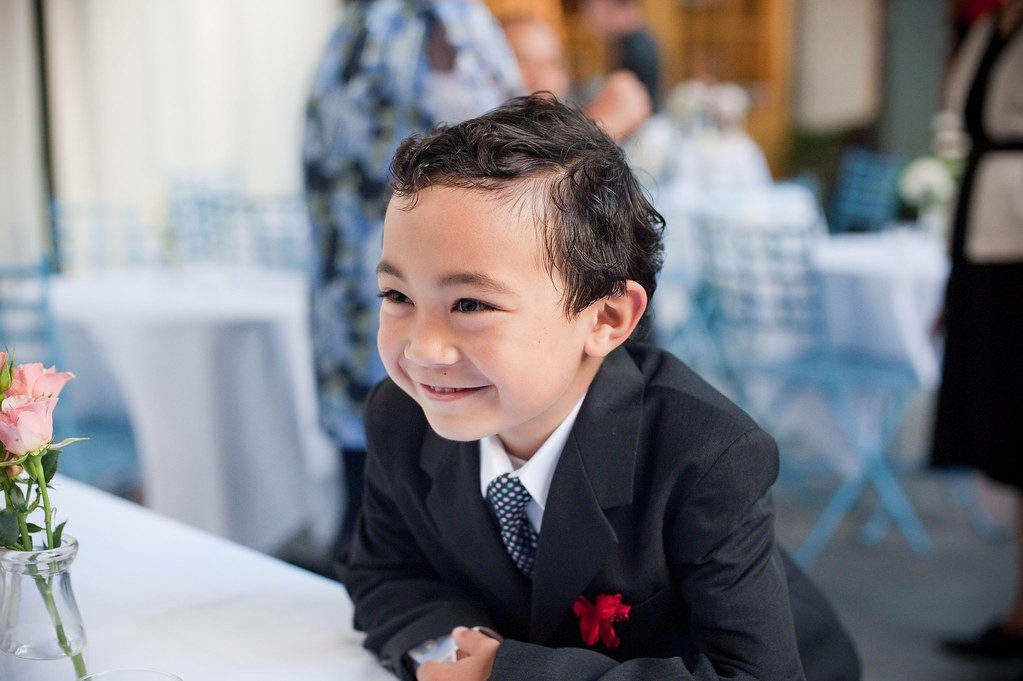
173;186;350;431
684;218;936;567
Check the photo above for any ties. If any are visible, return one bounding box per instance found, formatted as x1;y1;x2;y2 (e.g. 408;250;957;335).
488;474;540;587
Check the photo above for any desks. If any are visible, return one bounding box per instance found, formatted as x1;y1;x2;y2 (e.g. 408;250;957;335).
2;269;345;551
0;467;397;681
818;225;956;391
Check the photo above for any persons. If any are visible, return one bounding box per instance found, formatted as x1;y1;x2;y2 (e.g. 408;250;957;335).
305;0;663;575
927;0;1023;668
343;94;863;681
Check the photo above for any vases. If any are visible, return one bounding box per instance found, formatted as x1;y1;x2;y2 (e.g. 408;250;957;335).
0;533;88;681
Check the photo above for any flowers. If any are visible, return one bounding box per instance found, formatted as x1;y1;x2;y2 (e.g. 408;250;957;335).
573;594;632;651
0;343;90;549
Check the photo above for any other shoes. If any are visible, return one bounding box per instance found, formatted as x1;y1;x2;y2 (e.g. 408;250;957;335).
943;621;1023;667
